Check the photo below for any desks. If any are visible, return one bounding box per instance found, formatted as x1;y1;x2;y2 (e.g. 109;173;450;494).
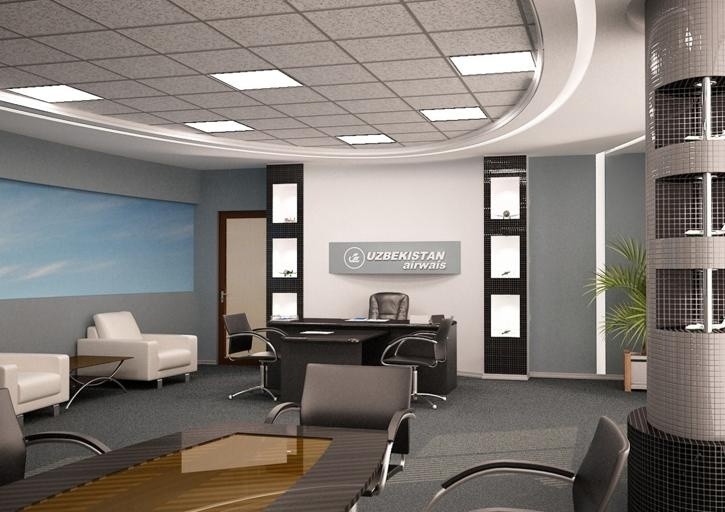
266;317;457;396
64;355;135;411
0;419;389;512
283;331;385;403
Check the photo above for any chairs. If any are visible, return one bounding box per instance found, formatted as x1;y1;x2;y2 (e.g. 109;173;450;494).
367;292;409;319
423;415;631;512
265;363;417;512
76;309;199;389
223;314;288;401
0;353;71;429
380;315;456;410
0;387;111;484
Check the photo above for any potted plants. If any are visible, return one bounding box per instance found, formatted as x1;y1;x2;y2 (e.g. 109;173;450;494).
581;235;648;393
